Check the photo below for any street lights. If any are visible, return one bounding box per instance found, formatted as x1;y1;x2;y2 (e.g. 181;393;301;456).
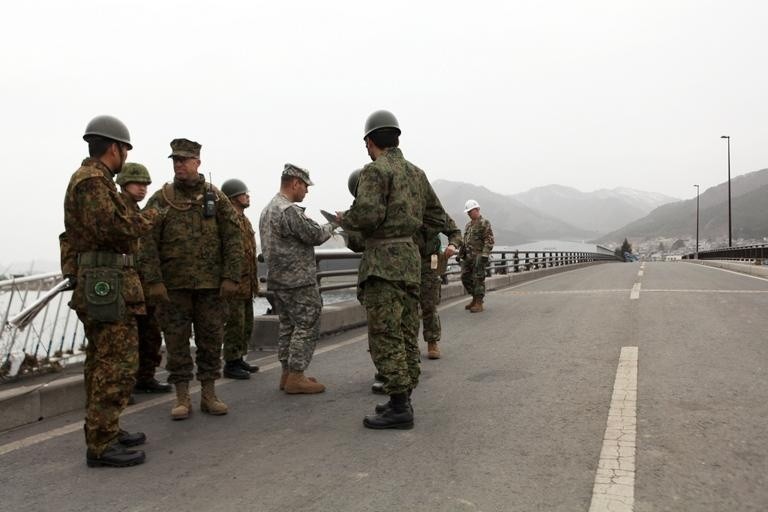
693;184;700;253
721;135;733;248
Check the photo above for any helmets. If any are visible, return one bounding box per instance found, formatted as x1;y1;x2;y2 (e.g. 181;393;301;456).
83;115;133;149
464;200;480;213
116;162;151;186
348;169;363;198
363;110;401;139
221;179;249;198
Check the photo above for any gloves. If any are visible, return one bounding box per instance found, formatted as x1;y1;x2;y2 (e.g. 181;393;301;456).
480;257;488;264
456;257;460;261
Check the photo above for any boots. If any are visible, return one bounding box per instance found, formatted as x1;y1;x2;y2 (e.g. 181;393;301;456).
280;367;318;390
363;370;414;430
428;340;441;359
465;295;476;309
285;371;325;394
470;295;483;312
86;356;258;468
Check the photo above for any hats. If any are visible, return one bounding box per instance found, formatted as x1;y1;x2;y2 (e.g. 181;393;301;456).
282;163;314;186
168;139;201;160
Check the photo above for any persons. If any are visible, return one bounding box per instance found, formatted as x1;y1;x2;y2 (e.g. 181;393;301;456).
57;115;148;468
340;108;445;431
258;163;335;397
414;211;464;360
140;137;246;424
335;168;387;394
457;198;496;315
114;161;175;407
220;177;260;381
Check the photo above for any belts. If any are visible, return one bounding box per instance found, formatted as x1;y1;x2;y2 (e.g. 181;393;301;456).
77;252;136;268
365;236;413;247
